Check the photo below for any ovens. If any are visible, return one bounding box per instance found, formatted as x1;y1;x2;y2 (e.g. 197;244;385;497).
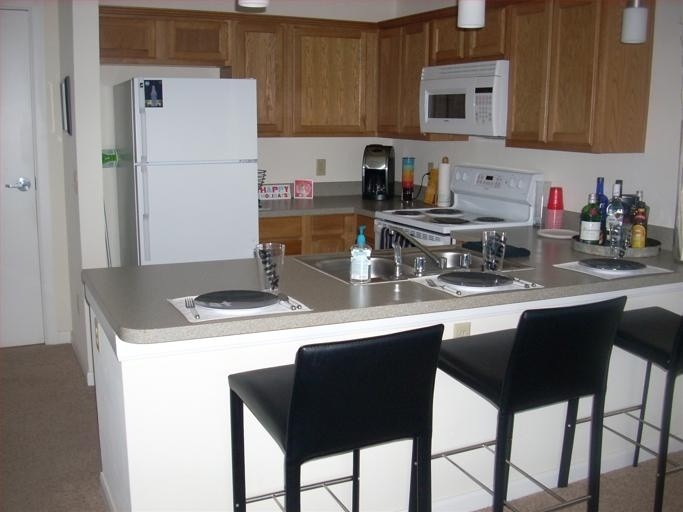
370;218;452;251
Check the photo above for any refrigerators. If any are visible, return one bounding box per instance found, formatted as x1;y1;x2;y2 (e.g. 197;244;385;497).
112;78;259;264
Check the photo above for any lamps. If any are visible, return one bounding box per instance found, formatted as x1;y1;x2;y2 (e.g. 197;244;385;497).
620;1;649;45
457;0;487;29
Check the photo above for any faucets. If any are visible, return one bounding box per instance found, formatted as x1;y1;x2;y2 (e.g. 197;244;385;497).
389;227;446;275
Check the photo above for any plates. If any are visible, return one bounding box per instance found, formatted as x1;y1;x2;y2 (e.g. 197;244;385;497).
578;258;646;271
438;271;514;288
195;290;278;310
537;228;579;239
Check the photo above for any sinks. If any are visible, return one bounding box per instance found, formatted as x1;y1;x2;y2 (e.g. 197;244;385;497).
291;246;436;287
393;244;535;273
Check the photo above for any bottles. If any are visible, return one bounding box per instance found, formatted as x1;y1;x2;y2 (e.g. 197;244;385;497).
630;208;647;249
604;184;624;246
578;193;603;245
636;190;650;223
594;176;609;247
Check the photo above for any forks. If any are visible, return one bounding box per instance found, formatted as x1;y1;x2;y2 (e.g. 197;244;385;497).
184;296;200;322
425;277;464;295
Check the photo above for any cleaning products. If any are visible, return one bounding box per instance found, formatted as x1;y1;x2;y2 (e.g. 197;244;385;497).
350;223;372;285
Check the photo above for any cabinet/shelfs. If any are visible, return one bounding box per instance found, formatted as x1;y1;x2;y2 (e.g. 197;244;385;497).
505;2;658;155
238;15;377;137
99;5;234;66
377;11;471;142
430;1;507;60
260;213;359;256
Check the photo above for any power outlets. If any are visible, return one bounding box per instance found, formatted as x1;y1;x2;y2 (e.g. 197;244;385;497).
258;182;292;200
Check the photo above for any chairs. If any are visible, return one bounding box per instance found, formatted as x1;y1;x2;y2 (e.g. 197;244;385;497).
560;305;682;512
435;294;628;512
230;323;444;512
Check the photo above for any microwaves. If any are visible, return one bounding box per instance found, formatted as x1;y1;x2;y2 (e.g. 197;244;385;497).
416;59;511;139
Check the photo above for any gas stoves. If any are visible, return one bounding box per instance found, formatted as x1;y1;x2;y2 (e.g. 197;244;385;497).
380;203;526;235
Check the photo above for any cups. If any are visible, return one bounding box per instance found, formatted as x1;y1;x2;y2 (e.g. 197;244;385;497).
548;185;564;210
482;230;508;273
252;241;286;294
609;221;634;259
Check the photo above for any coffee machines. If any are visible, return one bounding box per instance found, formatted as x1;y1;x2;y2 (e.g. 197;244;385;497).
361;142;395;201
